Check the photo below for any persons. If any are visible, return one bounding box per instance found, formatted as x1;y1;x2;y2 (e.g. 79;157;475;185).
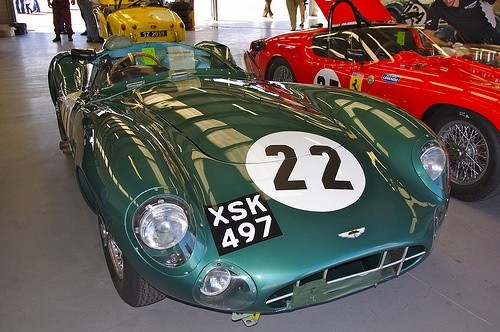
263;0;306;31
424;0;500;46
15;0;40;14
47;0;105;43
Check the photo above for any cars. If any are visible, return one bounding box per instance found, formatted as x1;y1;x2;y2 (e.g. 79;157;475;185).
92;0;186;44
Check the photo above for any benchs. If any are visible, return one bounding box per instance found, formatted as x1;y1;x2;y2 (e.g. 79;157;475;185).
93;41;212;82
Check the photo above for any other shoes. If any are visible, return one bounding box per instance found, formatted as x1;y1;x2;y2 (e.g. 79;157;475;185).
68;36;73;41
52;37;61;42
60;30;66;34
18;10;40;14
80;30;87;35
86;38;103;43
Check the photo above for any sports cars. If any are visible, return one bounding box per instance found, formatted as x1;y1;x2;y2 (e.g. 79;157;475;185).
46;31;453;327
242;0;500;204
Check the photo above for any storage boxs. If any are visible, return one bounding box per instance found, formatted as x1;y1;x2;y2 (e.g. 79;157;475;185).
0;24;17;37
